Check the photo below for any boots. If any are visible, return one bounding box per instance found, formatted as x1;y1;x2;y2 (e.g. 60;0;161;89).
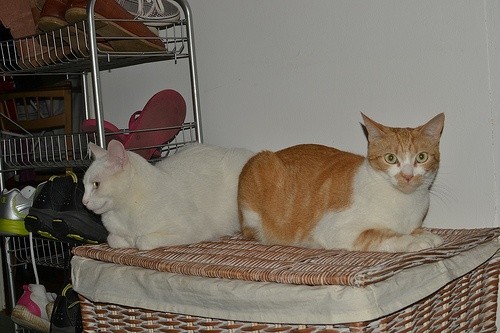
0;0;72;69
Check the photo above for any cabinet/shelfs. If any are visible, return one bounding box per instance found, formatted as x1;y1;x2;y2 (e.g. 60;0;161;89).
1;0;204;333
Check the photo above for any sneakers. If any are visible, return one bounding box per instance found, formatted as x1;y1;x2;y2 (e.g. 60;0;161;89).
10;283;57;333
115;0;185;26
0;181;36;236
24;169;109;244
50;283;83;333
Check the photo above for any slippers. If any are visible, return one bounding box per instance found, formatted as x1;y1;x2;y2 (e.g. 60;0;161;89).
82;119;161;159
124;89;186;158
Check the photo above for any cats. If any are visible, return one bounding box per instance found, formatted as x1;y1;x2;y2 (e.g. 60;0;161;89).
238;112;446;253
82;140;259;251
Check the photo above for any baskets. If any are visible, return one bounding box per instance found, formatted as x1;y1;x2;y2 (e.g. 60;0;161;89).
71;228;500;333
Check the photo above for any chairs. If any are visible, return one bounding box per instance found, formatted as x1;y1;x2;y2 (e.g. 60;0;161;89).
0;81;74;190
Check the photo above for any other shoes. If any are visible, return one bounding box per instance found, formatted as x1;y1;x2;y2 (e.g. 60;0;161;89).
65;0;166;54
39;0;113;57
